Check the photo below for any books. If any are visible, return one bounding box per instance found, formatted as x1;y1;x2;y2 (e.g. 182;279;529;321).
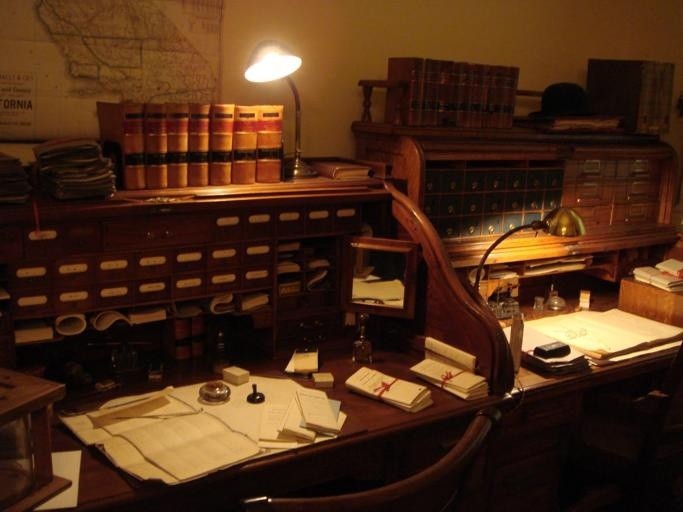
617;277;683;326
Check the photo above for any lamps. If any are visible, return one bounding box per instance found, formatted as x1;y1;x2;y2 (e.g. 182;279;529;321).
586;344;683;512
236;405;504;512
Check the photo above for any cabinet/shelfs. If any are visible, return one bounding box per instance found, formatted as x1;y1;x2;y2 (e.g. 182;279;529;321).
353;313;372;364
534;296;544;311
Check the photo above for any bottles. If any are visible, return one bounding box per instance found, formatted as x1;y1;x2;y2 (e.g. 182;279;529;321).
527;83;594;120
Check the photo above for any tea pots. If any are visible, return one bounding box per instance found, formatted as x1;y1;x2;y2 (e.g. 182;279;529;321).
343;235;419;320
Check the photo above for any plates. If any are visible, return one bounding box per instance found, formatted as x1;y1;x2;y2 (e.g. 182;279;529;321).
351;121;683;270
0;171;390;386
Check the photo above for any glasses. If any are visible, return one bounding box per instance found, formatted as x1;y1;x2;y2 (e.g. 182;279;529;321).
245;41;319;178
474;208;585;291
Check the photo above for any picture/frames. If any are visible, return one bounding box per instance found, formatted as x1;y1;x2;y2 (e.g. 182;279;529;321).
58;350;516;512
484;277;683;512
0;366;72;511
